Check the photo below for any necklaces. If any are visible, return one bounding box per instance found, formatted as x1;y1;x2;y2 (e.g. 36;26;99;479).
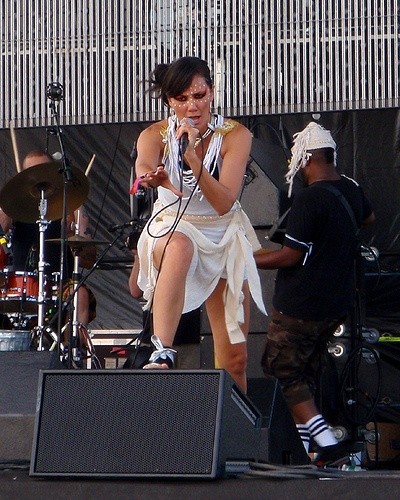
166;113;224;202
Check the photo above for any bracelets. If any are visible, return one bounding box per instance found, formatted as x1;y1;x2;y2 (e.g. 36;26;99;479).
140;164;165;188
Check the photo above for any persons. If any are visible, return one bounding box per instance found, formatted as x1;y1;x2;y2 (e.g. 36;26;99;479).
127;57;255;395
252;120;370;473
0;150;95;369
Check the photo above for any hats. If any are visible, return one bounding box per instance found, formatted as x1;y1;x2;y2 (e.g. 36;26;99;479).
285;122;336;197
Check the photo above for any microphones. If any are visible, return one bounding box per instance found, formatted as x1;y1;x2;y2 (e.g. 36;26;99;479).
108;221;134;233
47;82;64;108
180;119;195;152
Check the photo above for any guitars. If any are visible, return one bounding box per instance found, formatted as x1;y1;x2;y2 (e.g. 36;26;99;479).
253;228;286;255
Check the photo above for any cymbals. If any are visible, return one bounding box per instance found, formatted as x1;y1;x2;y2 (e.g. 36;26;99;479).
0;161;91;225
44;235;111;252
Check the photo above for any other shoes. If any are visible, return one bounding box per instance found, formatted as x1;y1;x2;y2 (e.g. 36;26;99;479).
313;442;349;468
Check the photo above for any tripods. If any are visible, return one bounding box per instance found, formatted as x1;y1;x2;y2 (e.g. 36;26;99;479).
29;189;103;369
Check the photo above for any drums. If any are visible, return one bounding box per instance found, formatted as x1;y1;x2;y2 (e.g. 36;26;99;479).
0;234;14;271
0;271;53;319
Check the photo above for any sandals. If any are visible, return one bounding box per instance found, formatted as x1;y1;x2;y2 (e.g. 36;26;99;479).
143;334;177;369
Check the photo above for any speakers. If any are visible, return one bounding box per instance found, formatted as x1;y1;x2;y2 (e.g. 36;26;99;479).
239;137;296;227
0;349;68;468
29;369;263;481
